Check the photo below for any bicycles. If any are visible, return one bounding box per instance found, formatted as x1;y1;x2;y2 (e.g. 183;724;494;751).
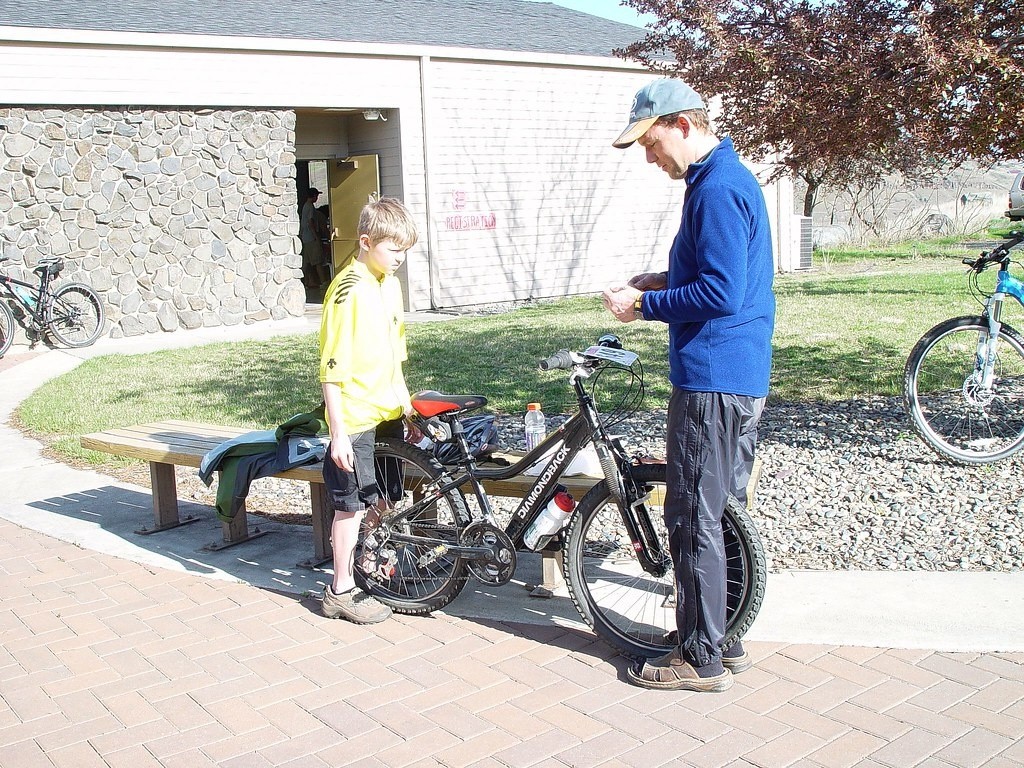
0;257;105;357
902;230;1024;467
352;334;767;664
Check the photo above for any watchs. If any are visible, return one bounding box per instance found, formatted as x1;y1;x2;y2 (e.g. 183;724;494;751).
632;291;646;321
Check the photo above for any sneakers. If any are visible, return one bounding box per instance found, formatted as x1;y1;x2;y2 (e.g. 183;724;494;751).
321;585;392;625
382;577;412;595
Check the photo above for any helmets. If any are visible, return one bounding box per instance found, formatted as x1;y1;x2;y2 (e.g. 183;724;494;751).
433;416;500;466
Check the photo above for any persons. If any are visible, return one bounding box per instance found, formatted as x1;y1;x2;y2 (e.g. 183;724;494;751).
318;189;420;628
300;188;331;288
600;75;777;694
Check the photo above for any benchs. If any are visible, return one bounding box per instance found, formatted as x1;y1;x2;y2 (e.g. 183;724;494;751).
82;418;764;600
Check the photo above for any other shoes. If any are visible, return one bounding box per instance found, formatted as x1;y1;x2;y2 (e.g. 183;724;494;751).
320;281;329;289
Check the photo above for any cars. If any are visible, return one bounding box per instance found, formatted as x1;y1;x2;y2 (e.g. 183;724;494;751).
1004;173;1024;222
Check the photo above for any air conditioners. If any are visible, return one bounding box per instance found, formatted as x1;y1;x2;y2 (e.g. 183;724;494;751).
791;212;813;270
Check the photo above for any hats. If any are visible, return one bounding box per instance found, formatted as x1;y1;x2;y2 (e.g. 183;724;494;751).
306;188;323;197
612;78;706;149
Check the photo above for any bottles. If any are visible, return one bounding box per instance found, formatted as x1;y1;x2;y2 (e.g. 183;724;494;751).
524;403;547;453
523;492;575;552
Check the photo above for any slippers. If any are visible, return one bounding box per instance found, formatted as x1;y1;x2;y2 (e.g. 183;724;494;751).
662;631;751;673
625;645;734;693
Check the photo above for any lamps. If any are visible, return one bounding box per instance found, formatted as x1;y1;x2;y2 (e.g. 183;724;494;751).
362;108;381;120
